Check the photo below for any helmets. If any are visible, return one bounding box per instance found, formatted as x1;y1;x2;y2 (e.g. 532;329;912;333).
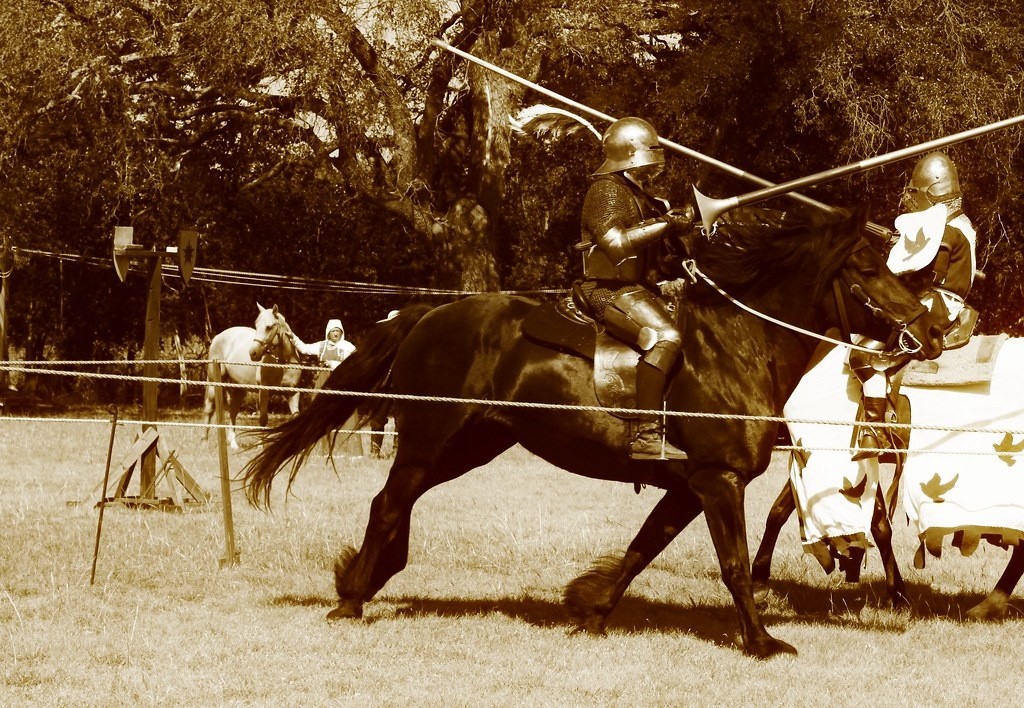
900;152;962;213
590;117;666;176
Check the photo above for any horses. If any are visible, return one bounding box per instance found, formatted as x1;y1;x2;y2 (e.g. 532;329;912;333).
204;205;1024;661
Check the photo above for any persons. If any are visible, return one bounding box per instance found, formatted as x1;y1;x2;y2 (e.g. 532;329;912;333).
285;320;364;458
578;117;696;461
849;151;977;461
369;311;400;459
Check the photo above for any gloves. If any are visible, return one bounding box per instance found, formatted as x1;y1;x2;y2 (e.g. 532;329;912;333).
661;203;697;238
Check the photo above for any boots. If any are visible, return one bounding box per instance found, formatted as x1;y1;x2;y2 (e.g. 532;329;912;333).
632;360;689;461
851;396;891;462
